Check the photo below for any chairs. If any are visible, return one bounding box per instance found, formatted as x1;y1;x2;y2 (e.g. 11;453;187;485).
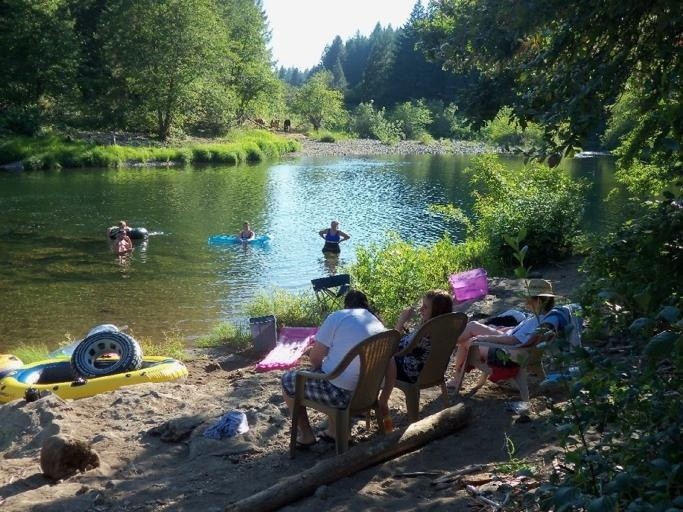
393;313;468;423
311;274;350;322
288;330;400;460
453;304;582;403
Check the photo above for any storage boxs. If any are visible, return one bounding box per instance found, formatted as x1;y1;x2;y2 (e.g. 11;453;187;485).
449;268;488;301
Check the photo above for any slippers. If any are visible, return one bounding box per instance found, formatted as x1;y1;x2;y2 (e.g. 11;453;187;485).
289;441;317;447
445;379;465;390
317;430;351;442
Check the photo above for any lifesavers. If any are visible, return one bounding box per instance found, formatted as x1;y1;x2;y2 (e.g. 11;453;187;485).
110;227;149;241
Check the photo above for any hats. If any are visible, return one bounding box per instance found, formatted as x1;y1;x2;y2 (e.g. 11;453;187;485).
522;279;561;298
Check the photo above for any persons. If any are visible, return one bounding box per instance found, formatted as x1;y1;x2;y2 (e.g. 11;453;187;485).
444;277;553;390
387;291;455;384
111;228;135;257
317;221;349;253
113;221;130;241
241;243;251;254
238;221;254;245
316;252;347;275
279;289;397;447
116;253;133;279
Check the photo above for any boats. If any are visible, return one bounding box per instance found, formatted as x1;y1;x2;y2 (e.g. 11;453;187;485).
209;233;273;244
0;354;188;403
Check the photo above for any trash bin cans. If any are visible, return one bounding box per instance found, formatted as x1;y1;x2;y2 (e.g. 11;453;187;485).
248;314;277;353
